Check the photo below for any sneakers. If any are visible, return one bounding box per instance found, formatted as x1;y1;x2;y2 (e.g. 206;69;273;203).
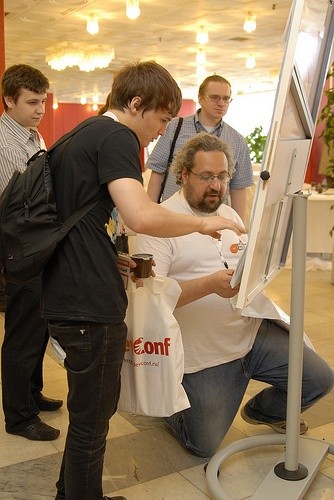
240;404;309;435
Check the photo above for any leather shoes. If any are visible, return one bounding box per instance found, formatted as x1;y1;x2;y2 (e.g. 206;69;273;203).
6;414;60;440
36;395;63;411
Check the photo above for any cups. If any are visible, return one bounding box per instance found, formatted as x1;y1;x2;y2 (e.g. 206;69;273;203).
130;253;154;278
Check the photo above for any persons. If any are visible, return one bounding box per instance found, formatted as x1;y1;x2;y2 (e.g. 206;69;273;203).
1;61;184;499
113;132;333;459
145;75;254;265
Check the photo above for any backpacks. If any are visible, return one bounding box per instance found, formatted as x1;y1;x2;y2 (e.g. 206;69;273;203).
0;115;115;282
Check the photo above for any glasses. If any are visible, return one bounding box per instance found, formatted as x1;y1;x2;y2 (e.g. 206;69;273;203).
187;168;232;185
203;94;233;103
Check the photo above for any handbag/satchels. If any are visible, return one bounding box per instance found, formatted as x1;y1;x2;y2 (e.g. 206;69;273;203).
117;274;191;418
